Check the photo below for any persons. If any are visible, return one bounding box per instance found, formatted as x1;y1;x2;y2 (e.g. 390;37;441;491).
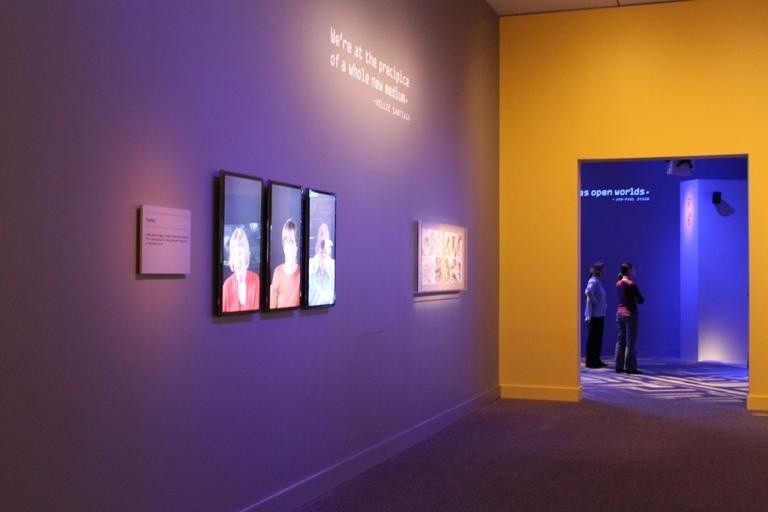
307;221;336;306
268;218;301;309
419;233;464;285
222;227;260;311
582;261;610;369
612;262;645;374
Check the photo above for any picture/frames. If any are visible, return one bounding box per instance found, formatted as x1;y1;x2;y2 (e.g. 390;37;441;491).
213;168;337;318
